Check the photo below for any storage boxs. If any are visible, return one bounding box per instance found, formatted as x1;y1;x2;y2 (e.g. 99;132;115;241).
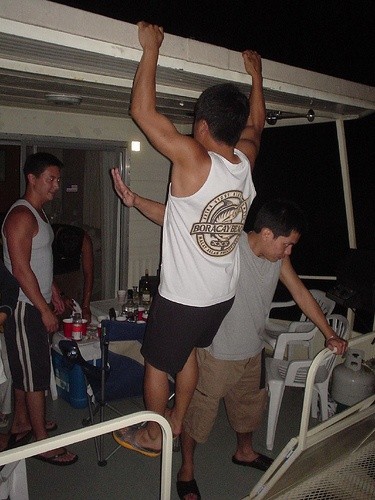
52;330;102;409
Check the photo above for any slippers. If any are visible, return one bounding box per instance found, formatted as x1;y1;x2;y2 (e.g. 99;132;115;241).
34;447;79;466
232;452;274;471
113;424;161;457
8;419;57;435
176;472;201;500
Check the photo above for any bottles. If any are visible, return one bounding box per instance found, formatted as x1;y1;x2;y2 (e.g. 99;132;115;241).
122;289;138;323
132;269;151;307
73;313;83;341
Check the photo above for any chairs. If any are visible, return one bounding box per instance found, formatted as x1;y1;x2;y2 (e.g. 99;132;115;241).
58;319;179;466
264;290;349;451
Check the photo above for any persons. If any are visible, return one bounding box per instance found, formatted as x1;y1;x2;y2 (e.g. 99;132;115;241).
111;167;349;500
111;20;267;459
0;151;95;466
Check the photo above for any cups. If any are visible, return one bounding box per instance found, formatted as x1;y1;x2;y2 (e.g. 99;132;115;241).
63;319;87;339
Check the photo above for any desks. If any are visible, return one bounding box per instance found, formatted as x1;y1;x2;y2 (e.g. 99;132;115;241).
90;298;147;328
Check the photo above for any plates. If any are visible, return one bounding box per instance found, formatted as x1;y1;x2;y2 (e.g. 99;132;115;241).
116;317;127;321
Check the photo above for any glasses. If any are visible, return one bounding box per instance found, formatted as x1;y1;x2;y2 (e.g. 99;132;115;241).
40;174;62;184
